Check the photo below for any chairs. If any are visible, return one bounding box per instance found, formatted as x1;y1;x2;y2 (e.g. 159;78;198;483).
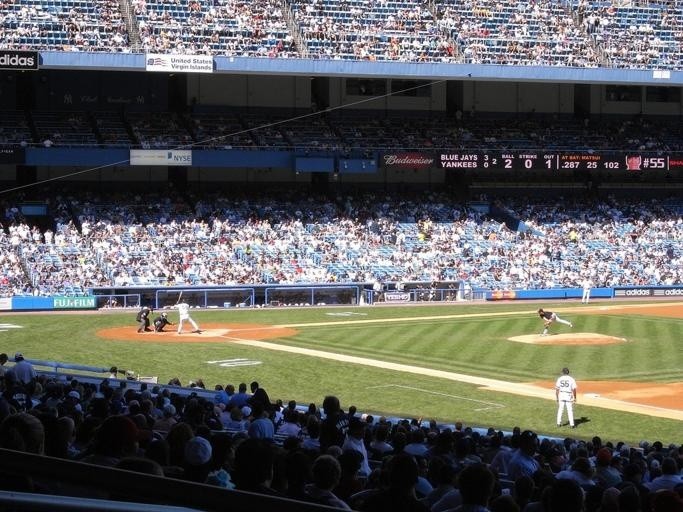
0;0;683;296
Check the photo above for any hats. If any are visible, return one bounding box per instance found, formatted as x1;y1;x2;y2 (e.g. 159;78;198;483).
69;391;80;399
596;448;612;461
241;406;252;416
184;437;212;464
16;352;24;358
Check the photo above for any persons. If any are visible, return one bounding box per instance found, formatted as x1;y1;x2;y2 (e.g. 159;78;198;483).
538;309;573;337
0;0;682;310
136;306;154;333
2;352;683;512
152;313;176;333
175;298;202;333
